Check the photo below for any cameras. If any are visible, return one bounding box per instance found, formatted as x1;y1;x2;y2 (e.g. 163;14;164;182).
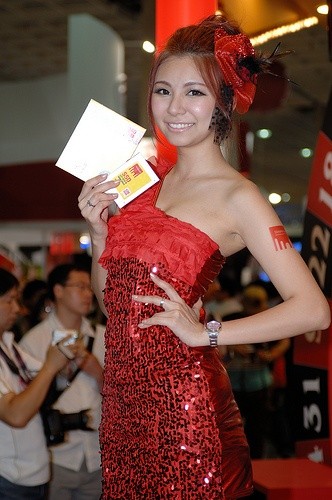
51;330;78;346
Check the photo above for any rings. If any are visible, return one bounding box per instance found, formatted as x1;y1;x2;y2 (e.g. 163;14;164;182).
160;297;165;307
87;200;95;207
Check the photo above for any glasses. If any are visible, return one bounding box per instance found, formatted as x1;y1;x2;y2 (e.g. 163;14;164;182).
64;281;92;290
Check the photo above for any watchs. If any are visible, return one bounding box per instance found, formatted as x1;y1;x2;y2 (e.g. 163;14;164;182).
205;319;221;348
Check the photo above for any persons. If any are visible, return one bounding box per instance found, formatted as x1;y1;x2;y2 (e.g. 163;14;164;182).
78;13;332;500
0;244;332;500
